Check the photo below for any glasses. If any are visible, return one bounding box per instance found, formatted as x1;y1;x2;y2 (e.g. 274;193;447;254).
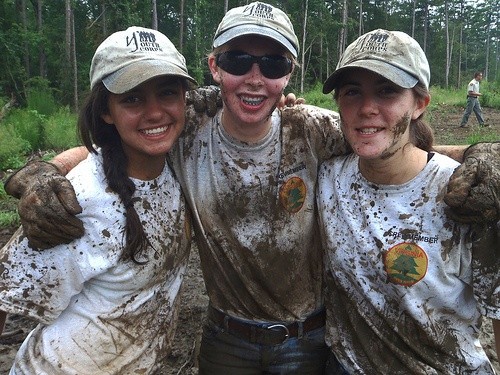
214;50;293;79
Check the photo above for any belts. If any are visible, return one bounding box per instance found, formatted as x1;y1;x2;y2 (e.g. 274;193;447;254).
207;304;327;347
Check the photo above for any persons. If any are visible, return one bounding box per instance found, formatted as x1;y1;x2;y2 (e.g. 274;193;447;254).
0;26;306;375
185;30;500;375
3;2;500;375
460;71;490;128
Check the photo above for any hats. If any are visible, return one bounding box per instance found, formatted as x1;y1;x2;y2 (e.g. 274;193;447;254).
322;28;430;95
212;1;301;59
90;26;198;96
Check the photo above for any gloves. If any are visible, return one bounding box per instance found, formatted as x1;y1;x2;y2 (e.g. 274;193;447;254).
5;160;87;250
441;142;500;227
